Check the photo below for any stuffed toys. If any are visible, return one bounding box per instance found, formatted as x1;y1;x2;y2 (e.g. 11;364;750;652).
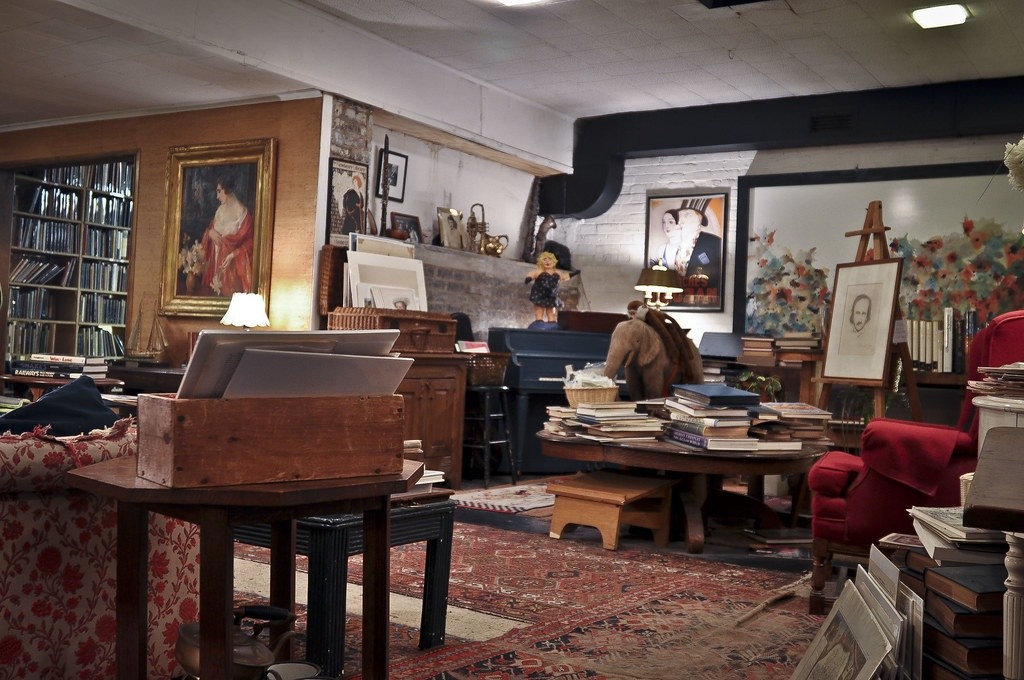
628;301;693;363
604;320;704;399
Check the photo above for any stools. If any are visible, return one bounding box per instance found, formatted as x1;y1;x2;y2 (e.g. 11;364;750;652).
232;500;455;678
546;472;673;551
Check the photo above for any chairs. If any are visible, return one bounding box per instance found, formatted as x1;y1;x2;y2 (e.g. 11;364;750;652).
808;310;1024;619
451;312;516;488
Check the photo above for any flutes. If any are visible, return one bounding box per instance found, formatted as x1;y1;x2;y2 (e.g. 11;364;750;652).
379;134;389;236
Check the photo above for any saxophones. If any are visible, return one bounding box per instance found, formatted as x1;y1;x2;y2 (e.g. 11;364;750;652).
523;176;557;264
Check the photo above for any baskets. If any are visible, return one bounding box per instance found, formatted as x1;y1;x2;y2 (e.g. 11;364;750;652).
563;387;619;408
467;352;511;386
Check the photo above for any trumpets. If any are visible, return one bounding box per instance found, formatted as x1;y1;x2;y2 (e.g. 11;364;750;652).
467;204;489;255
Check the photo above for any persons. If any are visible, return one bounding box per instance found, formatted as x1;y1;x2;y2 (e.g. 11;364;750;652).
525;252;569;324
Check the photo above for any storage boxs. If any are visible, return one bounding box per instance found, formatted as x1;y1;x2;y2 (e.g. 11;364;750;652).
461;352;512;386
137;392;404;487
320;245;457;352
326;148;465;311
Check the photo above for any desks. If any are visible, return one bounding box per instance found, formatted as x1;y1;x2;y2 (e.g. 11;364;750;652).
536;430;828;554
66;454;425;680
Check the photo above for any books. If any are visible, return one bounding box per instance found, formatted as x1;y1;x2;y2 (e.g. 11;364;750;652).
390;440;446;497
542;307;1024;680
0;163;138;414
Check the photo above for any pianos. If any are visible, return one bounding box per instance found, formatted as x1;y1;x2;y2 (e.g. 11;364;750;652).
487;328;645;481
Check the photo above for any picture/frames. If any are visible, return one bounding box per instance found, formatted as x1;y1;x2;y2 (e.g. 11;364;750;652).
157;137;278;318
789;580;893;680
811;257;904;388
732;159;1024;338
644;192;728;313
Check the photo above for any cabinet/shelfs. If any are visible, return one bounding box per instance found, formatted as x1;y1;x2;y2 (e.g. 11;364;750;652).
0;164;133;395
394;353;472;490
